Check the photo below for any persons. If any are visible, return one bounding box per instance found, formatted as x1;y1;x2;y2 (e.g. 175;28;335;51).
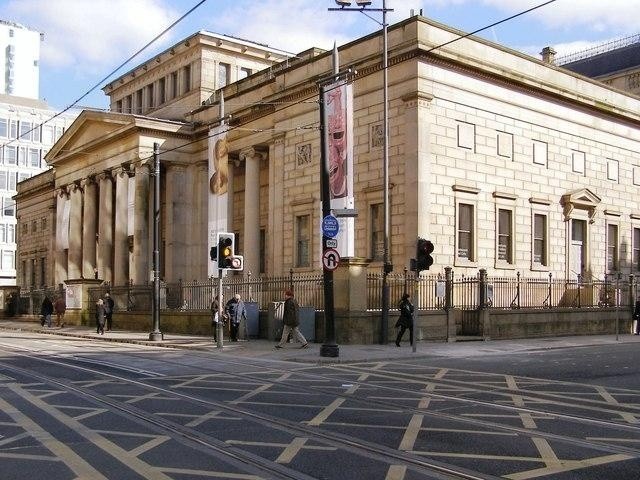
632;295;640;335
95;299;105;335
103;292;114;331
225;293;248;342
41;294;54;327
394;292;413;347
275;290;310;349
53;294;66;327
211;294;228;343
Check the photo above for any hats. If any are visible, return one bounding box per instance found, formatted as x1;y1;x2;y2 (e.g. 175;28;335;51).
286;291;293;296
234;294;240;300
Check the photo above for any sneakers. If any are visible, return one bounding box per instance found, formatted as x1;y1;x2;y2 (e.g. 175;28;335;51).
396;341;400;346
275;345;283;348
302;343;308;348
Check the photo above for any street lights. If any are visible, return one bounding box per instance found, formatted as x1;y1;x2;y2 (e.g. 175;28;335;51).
323;0;393;349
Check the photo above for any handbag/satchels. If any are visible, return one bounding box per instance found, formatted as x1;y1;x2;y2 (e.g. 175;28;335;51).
404;304;414;314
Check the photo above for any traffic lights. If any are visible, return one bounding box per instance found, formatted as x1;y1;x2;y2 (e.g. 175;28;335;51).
216;232;236;275
416;238;435;272
232;255;244;270
